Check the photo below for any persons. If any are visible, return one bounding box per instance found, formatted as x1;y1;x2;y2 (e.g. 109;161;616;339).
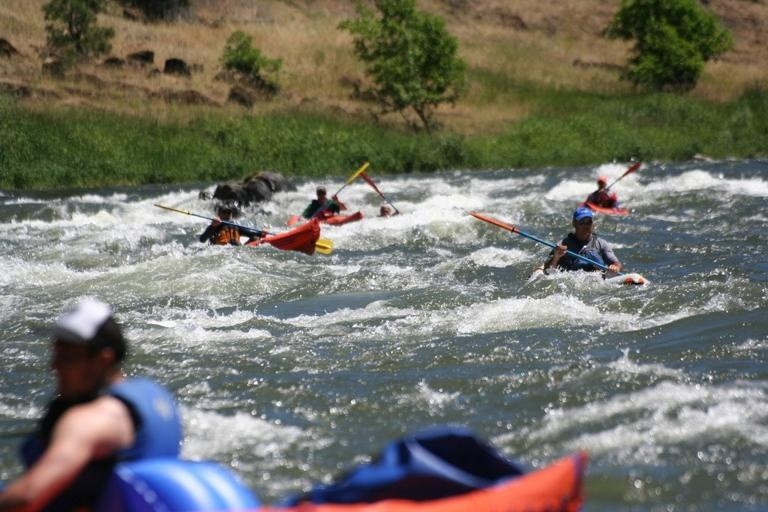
0;297;184;511
543;207;624;278
299;187;347;222
583;178;622;209
199;203;270;247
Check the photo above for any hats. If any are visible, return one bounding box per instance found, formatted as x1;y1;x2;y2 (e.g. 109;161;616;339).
317;186;326;194
573;207;594;222
218;205;232;214
598;176;608;186
32;295;127;366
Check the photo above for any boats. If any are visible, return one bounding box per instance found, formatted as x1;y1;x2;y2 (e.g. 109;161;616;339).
578;197;631;219
288;209;368;227
525;262;655;288
110;418;592;512
229;216;322;261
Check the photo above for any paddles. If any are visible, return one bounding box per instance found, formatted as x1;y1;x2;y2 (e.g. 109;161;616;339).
469;213;624;275
309;163;371;220
361;172;399;214
584;163;641;204
154;201;334;255
